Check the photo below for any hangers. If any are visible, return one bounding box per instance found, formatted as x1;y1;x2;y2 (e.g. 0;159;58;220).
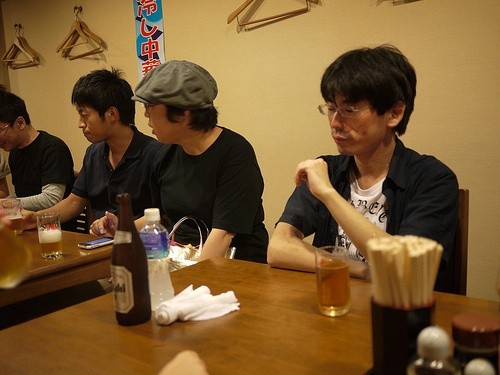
55;5;108;62
227;0;318;26
0;23;41;68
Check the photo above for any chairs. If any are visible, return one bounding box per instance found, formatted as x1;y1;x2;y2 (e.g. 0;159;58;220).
67;174;93;235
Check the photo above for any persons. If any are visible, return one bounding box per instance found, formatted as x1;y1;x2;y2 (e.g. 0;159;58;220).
90;59;270;263
265;42;460;291
0;91;76;233
3;66;162;232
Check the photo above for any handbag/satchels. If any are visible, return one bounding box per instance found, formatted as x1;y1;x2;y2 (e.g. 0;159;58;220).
167;215;235;275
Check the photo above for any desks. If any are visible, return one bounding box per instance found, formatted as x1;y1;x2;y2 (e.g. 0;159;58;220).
1;224;115;306
0;257;500;375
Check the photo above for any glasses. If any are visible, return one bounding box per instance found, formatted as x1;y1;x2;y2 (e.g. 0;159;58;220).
318;102;374;118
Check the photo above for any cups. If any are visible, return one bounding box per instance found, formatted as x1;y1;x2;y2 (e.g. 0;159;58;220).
0;220;31;288
0;197;24;236
315;246;352;317
365;295;437;375
36;210;64;261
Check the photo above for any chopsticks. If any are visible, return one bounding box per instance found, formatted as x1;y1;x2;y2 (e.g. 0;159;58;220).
366;234;443;309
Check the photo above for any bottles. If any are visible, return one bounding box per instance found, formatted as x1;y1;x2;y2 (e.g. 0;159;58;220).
451;310;500;375
407;325;461;375
464;358;496;375
110;194;151;327
139;207;175;312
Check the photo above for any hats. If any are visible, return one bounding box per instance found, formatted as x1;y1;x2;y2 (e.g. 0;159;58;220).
130;60;218;109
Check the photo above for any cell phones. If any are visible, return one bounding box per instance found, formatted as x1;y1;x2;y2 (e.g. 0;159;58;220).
77;236;114;250
